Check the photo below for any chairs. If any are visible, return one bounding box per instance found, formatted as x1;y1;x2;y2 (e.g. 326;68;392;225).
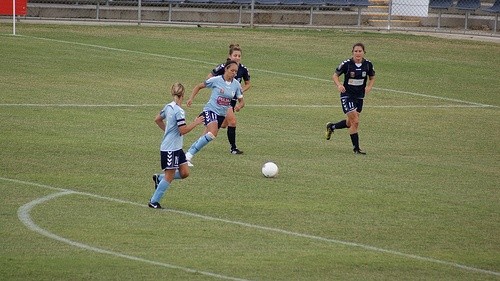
454;0;481;30
481;0;500;33
107;0;370;26
429;0;452;28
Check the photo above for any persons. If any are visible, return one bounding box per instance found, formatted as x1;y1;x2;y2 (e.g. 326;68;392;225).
207;44;251;153
147;83;205;209
183;57;245;167
325;42;375;155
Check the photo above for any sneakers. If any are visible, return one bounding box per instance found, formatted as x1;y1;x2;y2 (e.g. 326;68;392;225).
185;152;193;166
152;174;160;189
199;112;203;117
231;148;244;154
148;199;161;209
354;149;366;154
326;122;334;140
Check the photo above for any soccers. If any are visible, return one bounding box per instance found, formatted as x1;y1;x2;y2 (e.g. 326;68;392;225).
262;162;278;177
